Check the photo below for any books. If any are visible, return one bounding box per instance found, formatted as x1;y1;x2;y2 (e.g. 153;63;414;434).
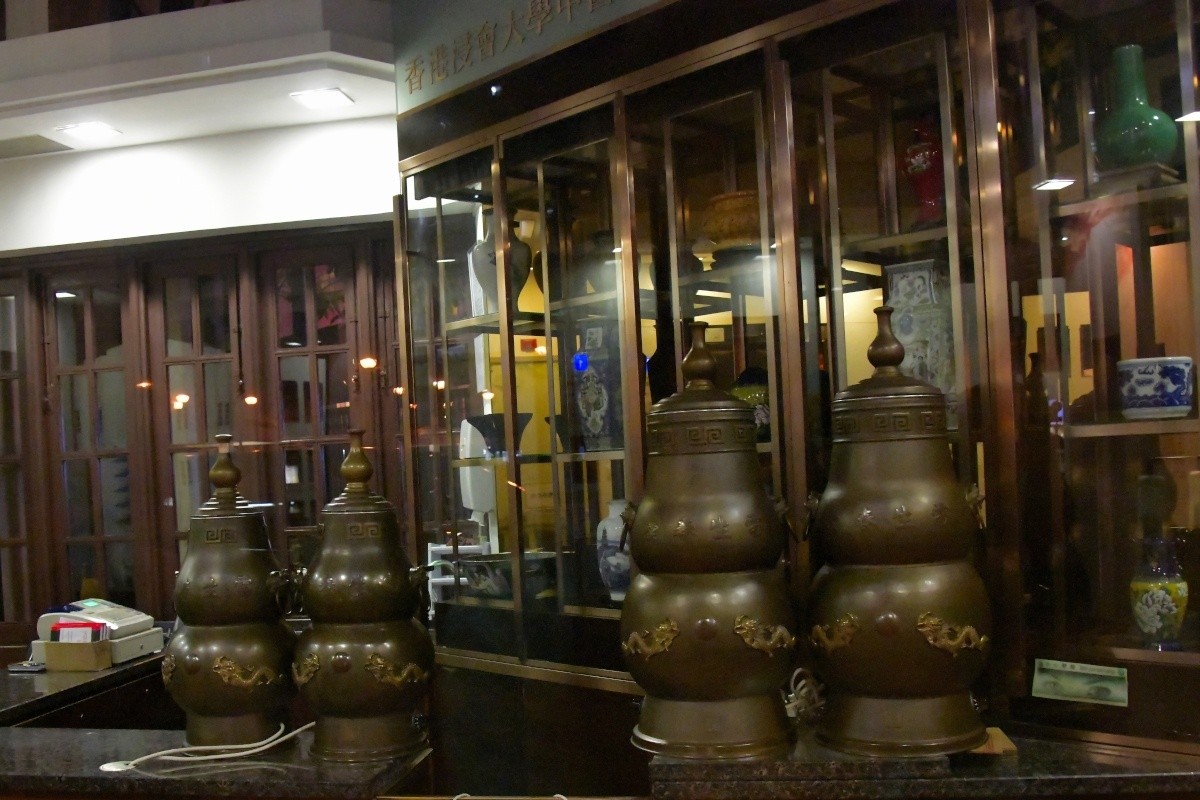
50;622;110;643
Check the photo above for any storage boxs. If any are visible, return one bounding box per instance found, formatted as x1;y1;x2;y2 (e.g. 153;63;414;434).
45;639;114;672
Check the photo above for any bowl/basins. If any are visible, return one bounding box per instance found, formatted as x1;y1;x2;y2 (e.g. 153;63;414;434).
458;551;573;598
704;190;775;249
466;411;534;459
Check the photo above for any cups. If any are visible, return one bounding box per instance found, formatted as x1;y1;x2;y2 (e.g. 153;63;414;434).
1116;357;1192;419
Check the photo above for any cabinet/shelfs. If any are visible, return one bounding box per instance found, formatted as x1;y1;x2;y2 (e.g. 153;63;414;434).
391;0;1200;759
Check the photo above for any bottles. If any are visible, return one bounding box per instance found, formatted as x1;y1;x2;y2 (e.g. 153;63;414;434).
161;432;295;760
572;313;621;450
802;305;990;757
1129;535;1189;653
1095;43;1183;173
472;207;531;313
616;320;796;763
595;500;633;601
289;428;434;765
887;260;963;431
904;118;946;232
584;232;640;291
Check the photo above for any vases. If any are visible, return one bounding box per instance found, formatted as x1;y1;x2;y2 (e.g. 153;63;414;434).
575;343;630;452
882;260;971;433
895;105;968;230
1127;534;1192;653
467;207;531;317
1088;42;1177;170
596;497;636;604
728;349;773;445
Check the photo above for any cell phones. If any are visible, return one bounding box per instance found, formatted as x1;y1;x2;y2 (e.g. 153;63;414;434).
8;665;47;672
